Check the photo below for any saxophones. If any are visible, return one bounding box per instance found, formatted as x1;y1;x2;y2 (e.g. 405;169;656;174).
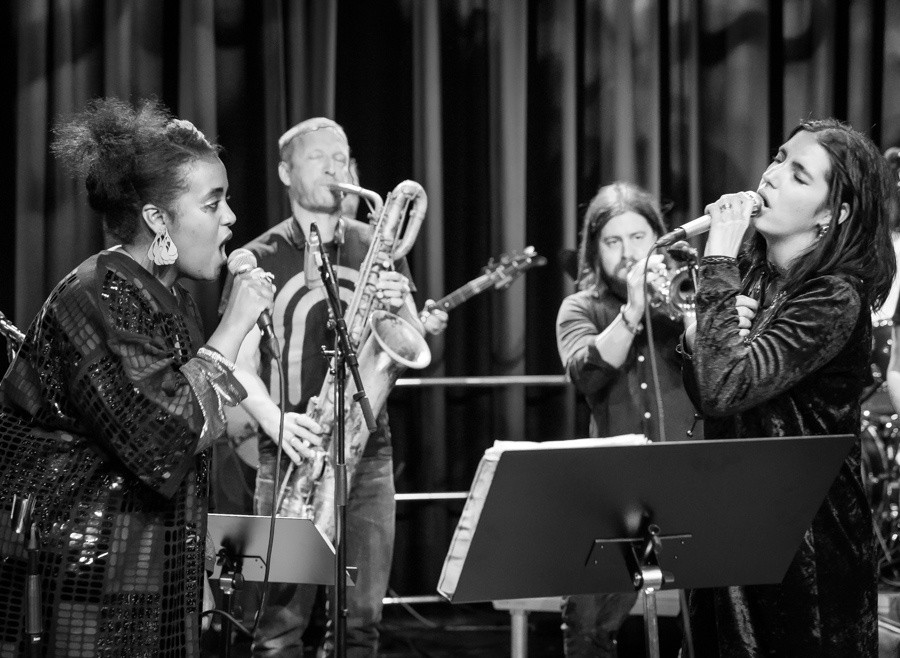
268;178;433;545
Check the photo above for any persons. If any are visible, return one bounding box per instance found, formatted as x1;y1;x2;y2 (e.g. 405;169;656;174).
555;111;899;658
218;120;449;658
0;94;280;658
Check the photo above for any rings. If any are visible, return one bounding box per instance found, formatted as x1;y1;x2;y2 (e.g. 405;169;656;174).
721;203;732;208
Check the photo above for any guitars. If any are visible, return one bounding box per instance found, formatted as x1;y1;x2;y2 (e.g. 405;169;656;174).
221;243;547;470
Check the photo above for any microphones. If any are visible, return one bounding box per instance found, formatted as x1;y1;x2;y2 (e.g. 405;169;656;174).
304;222;324;288
669;240;698;262
655;190;763;248
227;248;281;359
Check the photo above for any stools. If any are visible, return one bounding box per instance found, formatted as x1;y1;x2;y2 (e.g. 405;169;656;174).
491;588;702;658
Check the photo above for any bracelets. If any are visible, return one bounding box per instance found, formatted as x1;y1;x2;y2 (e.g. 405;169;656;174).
702;257;737;266
199;345;235;372
619;305;646;333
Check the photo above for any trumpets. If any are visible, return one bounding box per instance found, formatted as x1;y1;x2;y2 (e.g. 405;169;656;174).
624;258;699;318
